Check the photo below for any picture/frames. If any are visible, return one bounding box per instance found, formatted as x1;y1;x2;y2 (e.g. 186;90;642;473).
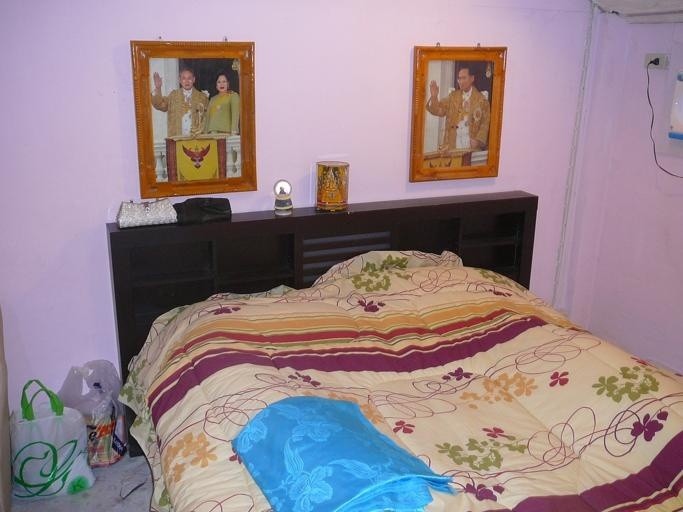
129;41;257;199
410;44;507;183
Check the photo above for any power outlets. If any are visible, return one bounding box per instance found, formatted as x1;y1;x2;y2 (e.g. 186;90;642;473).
644;53;668;69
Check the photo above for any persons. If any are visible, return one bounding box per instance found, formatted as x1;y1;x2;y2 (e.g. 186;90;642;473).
193;72;240;136
151;67;210;138
425;64;490;153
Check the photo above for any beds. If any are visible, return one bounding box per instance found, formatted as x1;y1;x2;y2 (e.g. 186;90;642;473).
106;191;683;512
